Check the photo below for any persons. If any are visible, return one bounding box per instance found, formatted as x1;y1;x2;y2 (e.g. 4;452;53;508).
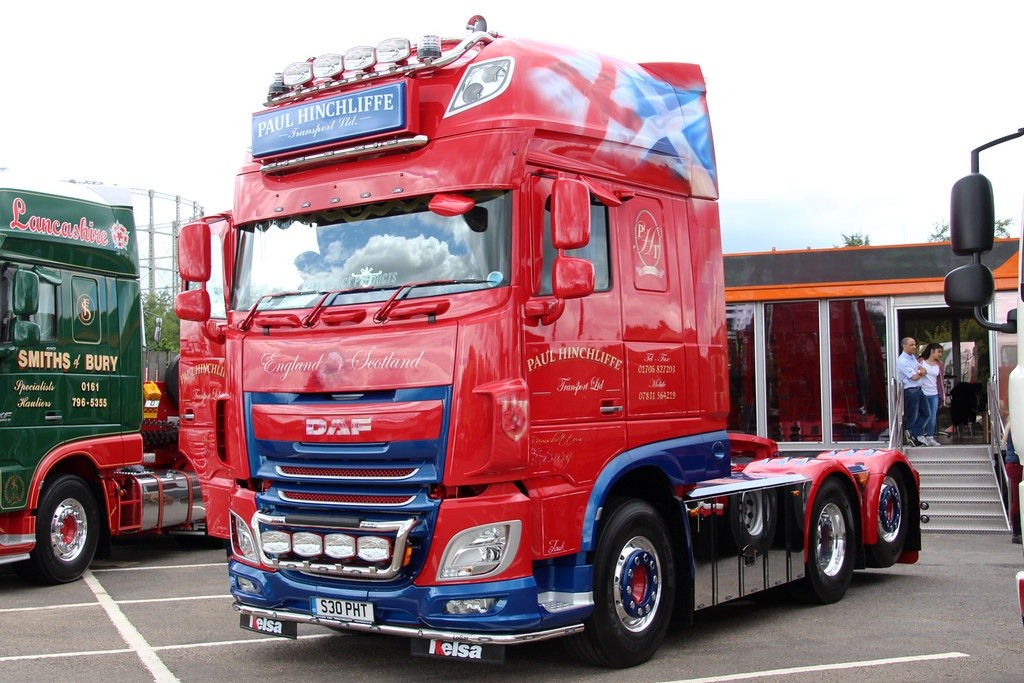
894;337;932;446
914;341;945;447
294;251;325;290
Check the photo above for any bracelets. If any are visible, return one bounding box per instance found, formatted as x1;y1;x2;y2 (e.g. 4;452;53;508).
917;370;920;374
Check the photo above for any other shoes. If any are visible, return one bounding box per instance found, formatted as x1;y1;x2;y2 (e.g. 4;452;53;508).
917;436;929;446
926;439;940;446
904;430;917;446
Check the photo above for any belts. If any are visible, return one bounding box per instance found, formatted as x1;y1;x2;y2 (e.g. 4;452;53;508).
904;387;922;391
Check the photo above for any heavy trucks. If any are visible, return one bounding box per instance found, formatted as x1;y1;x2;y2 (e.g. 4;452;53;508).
174;14;933;668
0;185;217;584
941;124;1024;627
